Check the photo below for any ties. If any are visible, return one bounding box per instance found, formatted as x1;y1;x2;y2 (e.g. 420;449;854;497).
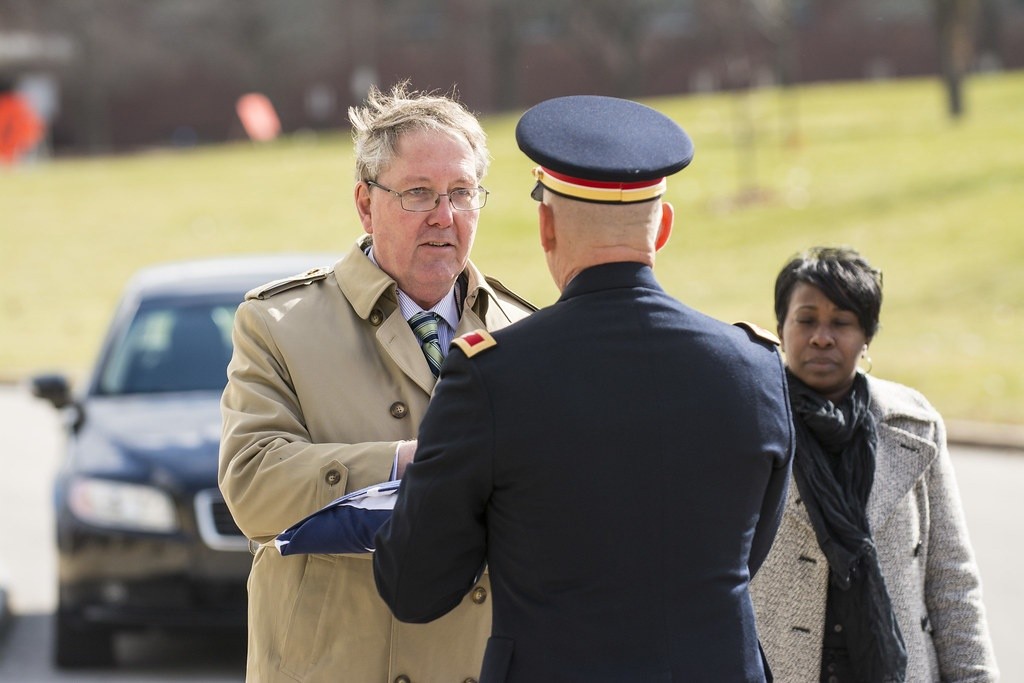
408;311;450;383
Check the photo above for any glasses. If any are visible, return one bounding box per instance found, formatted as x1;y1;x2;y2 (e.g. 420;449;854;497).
367;180;491;212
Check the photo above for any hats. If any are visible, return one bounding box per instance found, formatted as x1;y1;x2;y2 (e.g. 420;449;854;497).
515;95;694;204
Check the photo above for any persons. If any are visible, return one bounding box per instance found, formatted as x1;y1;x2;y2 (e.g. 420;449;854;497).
219;93;538;683
748;250;999;683
374;96;793;682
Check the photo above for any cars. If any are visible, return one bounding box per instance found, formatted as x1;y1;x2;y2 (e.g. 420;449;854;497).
24;252;353;671
